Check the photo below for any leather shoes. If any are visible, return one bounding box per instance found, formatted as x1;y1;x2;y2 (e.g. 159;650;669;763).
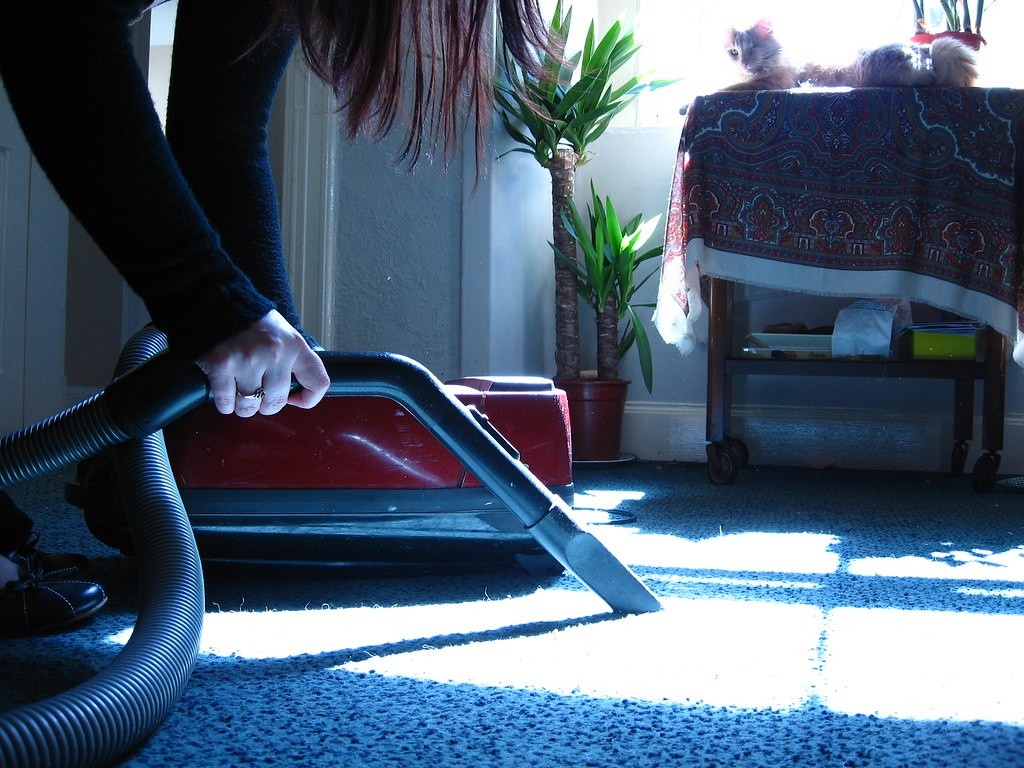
6;530;88;580
0;569;108;636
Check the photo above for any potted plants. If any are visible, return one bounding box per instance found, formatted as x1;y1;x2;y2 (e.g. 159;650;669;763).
910;0;987;51
486;0;664;462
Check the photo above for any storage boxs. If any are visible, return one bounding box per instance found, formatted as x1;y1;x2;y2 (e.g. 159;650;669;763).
913;329;978;360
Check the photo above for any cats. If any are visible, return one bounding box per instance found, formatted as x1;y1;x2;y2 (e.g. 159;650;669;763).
679;17;979;116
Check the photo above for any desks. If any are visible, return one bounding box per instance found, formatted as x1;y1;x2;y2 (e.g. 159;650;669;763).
652;84;1024;493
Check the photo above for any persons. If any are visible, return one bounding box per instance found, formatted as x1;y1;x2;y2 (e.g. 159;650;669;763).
0;0;552;629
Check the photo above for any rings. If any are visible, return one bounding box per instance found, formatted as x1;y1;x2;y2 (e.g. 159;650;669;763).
237;388;265;400
